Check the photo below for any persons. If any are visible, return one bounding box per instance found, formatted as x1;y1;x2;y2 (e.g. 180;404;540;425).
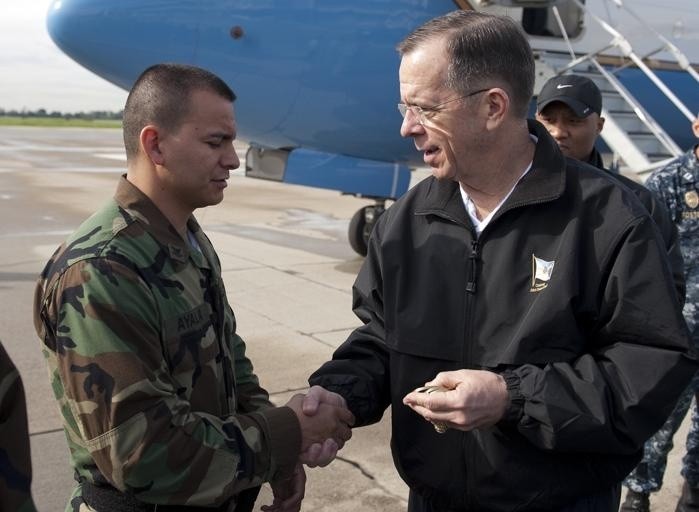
533;73;687;316
34;58;356;512
300;8;698;512
618;113;697;511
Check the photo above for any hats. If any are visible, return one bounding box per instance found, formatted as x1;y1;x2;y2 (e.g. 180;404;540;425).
536;74;602;120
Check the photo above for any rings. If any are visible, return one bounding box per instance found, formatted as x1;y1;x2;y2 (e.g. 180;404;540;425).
433;422;451;434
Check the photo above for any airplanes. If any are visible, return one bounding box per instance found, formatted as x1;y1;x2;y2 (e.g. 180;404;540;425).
38;0;697;260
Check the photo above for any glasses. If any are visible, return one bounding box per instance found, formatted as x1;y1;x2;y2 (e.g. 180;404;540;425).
395;86;490;122
